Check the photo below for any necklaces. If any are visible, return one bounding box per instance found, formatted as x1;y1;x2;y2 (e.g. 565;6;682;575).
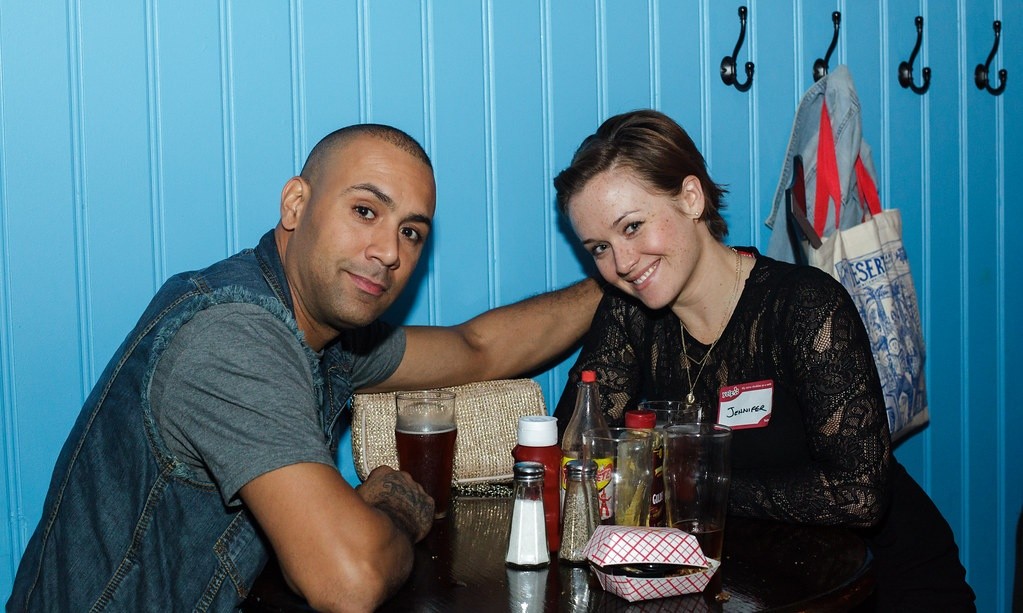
680;243;741;411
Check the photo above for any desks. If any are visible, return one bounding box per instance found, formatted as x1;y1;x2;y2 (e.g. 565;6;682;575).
245;499;877;613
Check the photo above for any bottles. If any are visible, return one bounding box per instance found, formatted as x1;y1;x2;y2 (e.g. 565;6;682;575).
511;417;563;555
562;370;616;526
620;409;667;529
559;460;603;565
504;457;550;569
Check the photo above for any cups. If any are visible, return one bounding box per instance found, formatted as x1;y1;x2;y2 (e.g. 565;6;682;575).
662;421;731;563
638;401;702;520
394;389;458;520
580;427;651;528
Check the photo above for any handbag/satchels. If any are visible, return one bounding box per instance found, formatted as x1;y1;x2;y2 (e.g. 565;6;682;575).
350;377;547;499
807;97;930;445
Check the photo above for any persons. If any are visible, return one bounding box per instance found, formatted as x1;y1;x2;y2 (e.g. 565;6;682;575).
5;122;611;613
550;109;978;612
